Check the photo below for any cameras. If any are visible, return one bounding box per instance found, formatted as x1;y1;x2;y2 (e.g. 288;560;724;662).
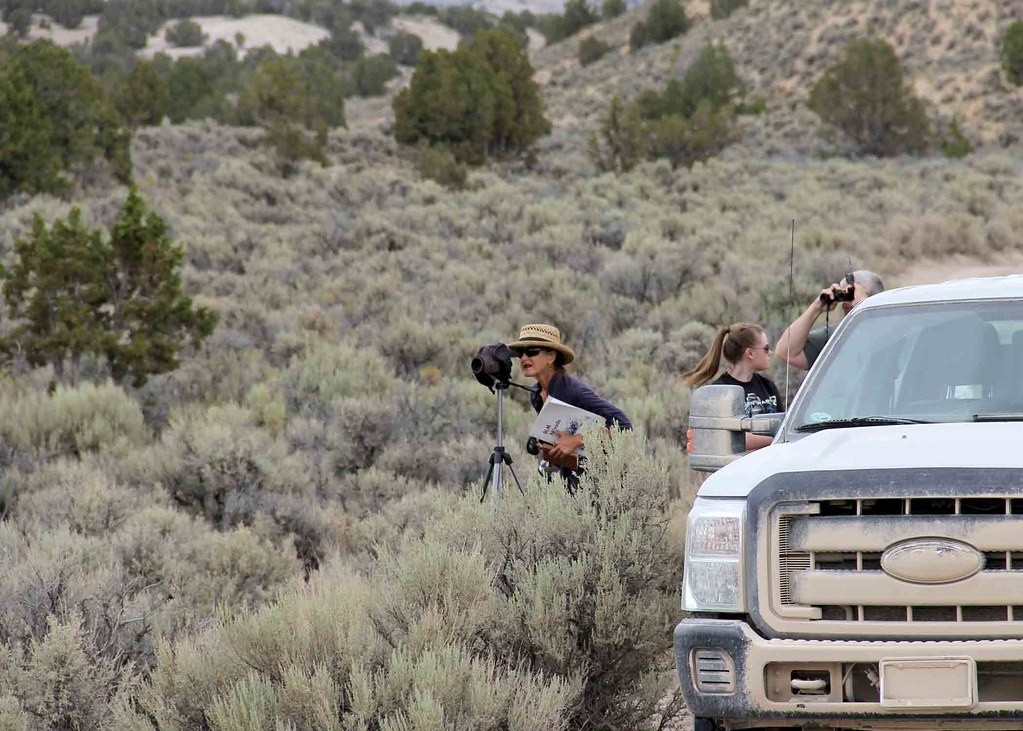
471;342;512;379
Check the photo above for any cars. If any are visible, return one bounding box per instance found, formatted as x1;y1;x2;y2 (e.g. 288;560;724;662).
672;276;1023;731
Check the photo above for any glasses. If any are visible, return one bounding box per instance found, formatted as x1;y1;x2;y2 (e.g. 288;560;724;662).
750;344;771;354
516;347;548;359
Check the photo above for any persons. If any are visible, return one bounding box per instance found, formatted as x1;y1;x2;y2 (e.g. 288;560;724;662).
504;323;633;513
777;270;905;424
682;324;785;453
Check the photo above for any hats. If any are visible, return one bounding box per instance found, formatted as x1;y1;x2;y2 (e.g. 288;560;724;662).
507;324;575;365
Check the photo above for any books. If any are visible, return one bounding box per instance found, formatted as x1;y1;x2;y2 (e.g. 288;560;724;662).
529;395;606;458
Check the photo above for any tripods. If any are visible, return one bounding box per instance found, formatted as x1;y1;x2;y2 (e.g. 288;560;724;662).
479;376;540;504
